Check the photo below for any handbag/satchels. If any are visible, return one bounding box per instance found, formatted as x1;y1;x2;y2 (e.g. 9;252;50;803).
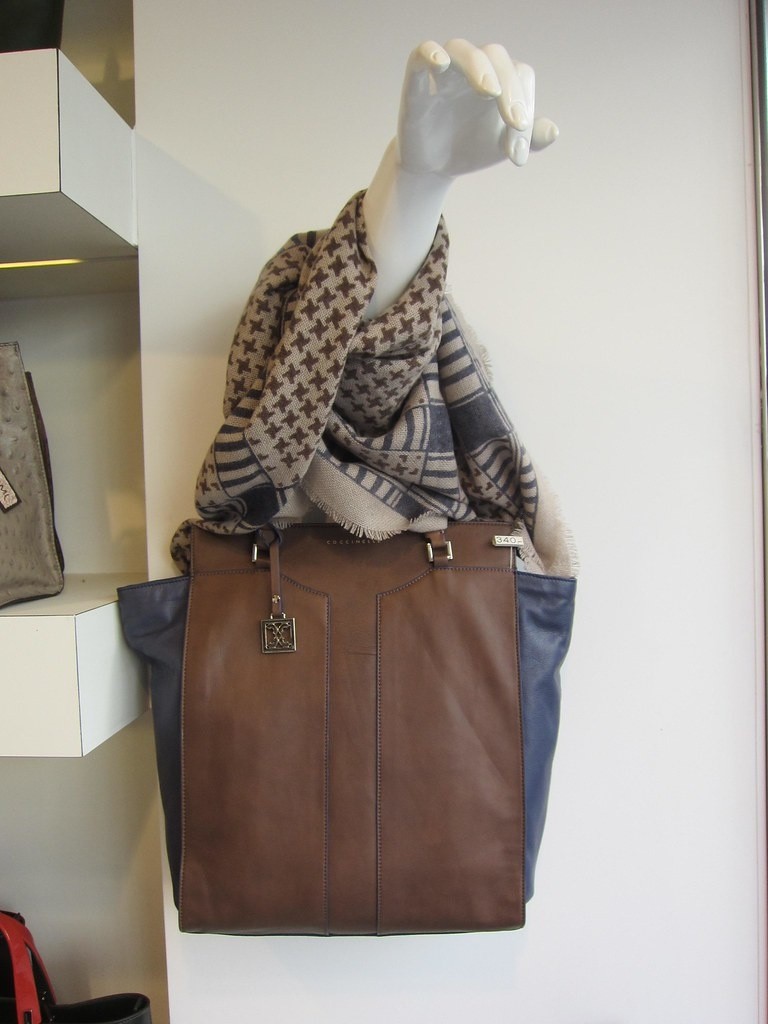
0;342;69;609
115;518;575;937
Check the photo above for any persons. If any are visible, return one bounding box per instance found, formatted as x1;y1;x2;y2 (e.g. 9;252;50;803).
173;30;569;565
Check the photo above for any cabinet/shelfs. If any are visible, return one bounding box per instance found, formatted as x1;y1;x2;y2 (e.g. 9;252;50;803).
1;50;149;759
